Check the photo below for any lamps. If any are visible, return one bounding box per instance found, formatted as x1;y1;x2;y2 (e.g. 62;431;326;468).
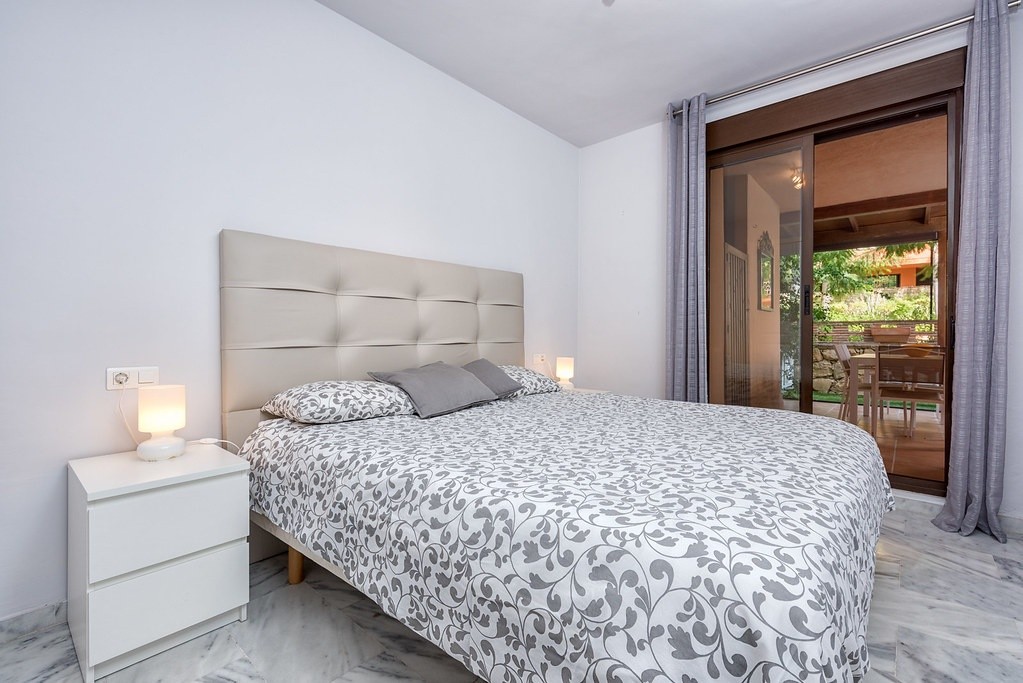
789;166;802;190
136;384;186;461
556;357;575;387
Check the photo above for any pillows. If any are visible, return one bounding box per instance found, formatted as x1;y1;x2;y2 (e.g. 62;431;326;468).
497;364;563;399
464;357;525;399
260;380;416;424
365;360;498;420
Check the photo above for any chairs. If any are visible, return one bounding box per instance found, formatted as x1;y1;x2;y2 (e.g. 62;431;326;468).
872;345;946;443
832;343;907;437
908;366;944;440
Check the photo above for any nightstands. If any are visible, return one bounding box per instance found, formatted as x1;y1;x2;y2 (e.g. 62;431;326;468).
573;388;612;393
66;439;251;683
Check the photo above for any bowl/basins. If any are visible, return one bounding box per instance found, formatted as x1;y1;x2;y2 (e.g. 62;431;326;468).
904;348;932;358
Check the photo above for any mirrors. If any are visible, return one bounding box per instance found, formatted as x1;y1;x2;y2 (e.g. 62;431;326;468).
757;230;775;313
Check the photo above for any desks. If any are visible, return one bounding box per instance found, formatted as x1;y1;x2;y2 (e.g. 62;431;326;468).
849;353;944;429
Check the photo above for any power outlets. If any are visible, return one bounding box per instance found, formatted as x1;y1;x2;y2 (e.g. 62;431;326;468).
106;366;161;391
533;354;545;364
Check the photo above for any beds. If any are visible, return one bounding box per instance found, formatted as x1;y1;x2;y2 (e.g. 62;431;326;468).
218;228;897;683
